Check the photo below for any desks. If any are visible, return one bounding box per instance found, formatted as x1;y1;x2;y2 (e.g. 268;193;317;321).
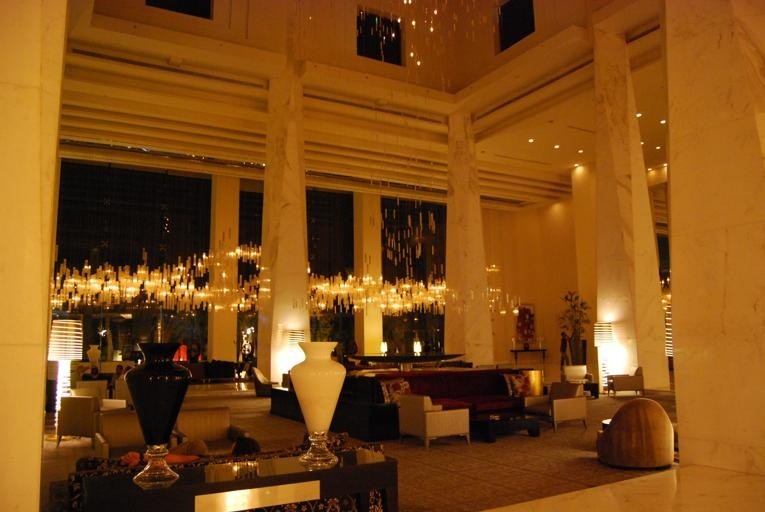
84;441;400;512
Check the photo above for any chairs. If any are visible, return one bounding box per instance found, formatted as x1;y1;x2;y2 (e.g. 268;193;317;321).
397;393;471;447
251;366;277;396
560;363;599;400
596;396;675;469
56;378;133;449
607;366;643;396
521;382;588;434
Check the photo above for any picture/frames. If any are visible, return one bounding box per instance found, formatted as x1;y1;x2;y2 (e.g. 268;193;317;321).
512;302;537;344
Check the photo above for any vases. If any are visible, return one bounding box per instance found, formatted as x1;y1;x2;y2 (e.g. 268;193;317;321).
123;341;193;491
288;342;346;471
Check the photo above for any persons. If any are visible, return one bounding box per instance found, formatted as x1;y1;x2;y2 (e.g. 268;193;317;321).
109;364;123;385
120;365;134;381
85;367;107;381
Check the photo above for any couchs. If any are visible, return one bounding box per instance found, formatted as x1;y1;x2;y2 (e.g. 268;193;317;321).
92;408;260;461
270;354;548;443
